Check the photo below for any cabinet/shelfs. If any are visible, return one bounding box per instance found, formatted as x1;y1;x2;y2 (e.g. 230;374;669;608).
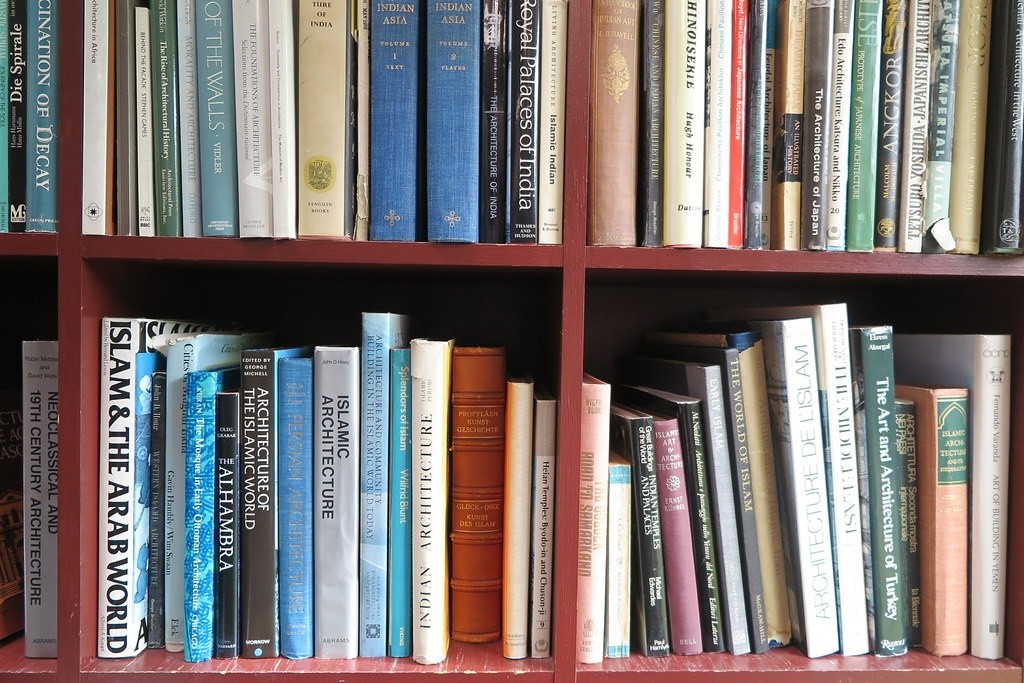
0;0;1024;683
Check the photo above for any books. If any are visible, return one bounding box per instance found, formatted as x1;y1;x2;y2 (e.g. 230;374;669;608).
0;301;1022;661
0;0;1024;259
